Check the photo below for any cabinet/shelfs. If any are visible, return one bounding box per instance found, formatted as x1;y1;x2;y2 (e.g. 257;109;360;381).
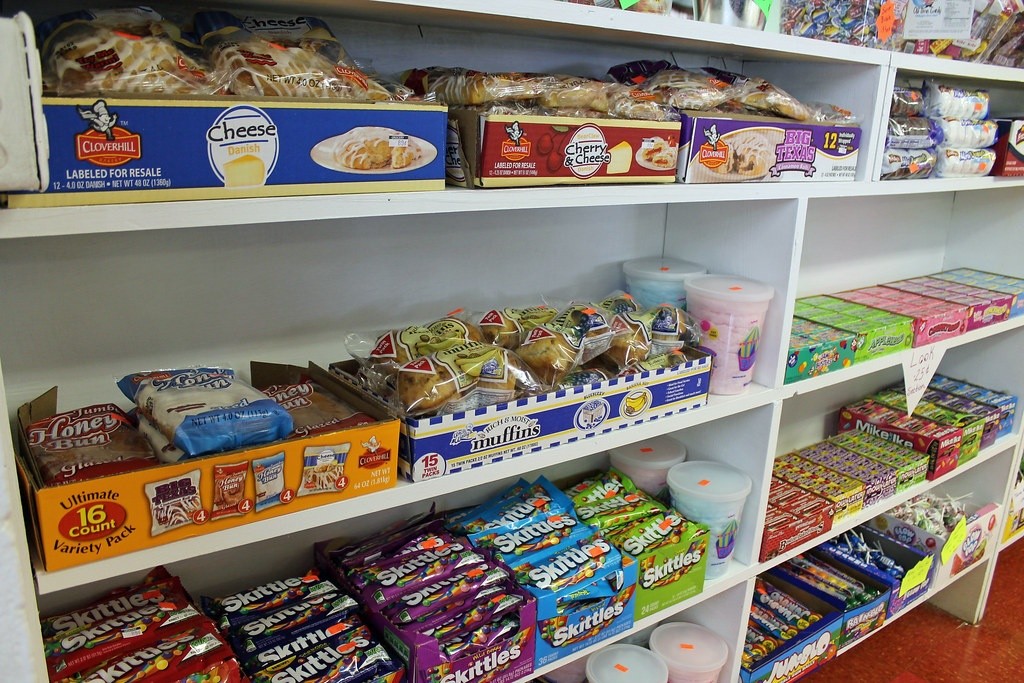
0;0;1024;683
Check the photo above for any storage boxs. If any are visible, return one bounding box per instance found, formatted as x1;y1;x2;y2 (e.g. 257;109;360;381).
15;268;1023;683
995;118;1024;177
7;95;863;193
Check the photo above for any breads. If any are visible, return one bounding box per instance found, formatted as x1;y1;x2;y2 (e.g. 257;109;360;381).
363;292;691;416
707;133;772;177
643;141;678;168
39;4;816;122
333;125;423;170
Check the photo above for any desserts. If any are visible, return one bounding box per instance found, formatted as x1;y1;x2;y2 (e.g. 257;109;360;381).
605;141;633;174
223;154;264;187
